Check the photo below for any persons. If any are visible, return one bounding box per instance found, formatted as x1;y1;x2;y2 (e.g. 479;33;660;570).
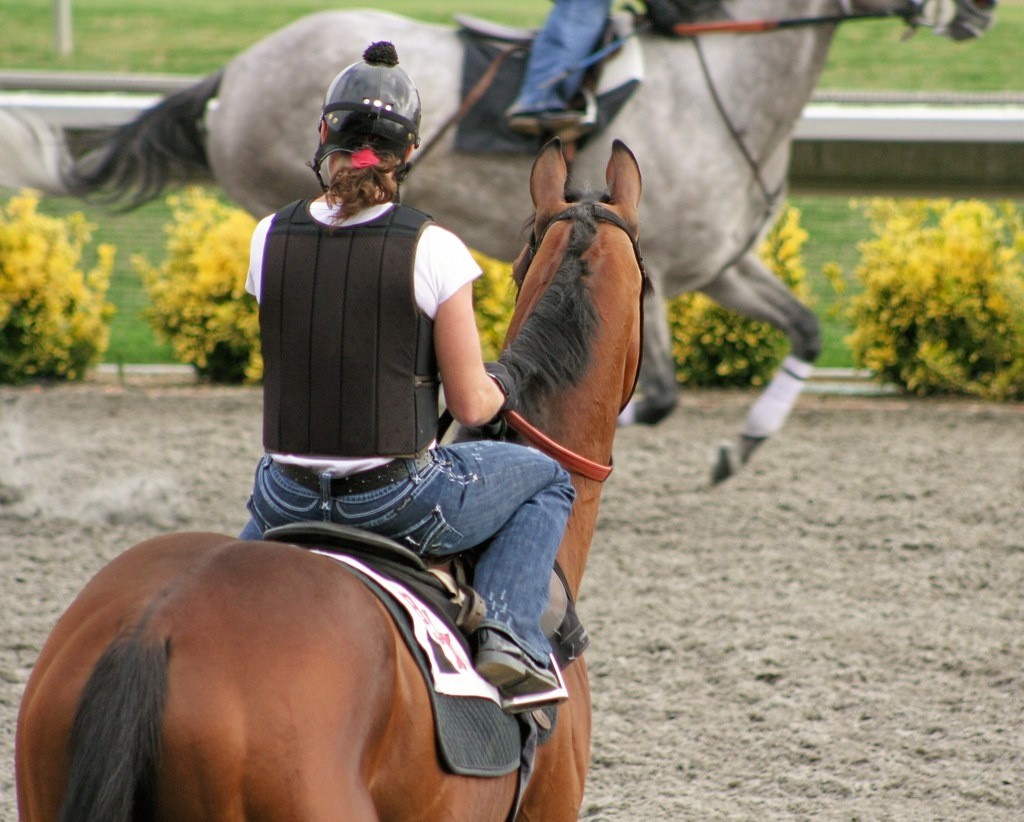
502;0;613;138
235;41;577;701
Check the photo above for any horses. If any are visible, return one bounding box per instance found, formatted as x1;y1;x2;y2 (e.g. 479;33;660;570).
10;137;658;821
2;0;1001;497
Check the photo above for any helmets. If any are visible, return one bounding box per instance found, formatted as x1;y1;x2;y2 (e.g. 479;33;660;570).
315;59;421;160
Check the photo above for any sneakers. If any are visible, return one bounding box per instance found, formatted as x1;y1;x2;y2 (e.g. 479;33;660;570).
474;629;560;696
511;107;588;139
